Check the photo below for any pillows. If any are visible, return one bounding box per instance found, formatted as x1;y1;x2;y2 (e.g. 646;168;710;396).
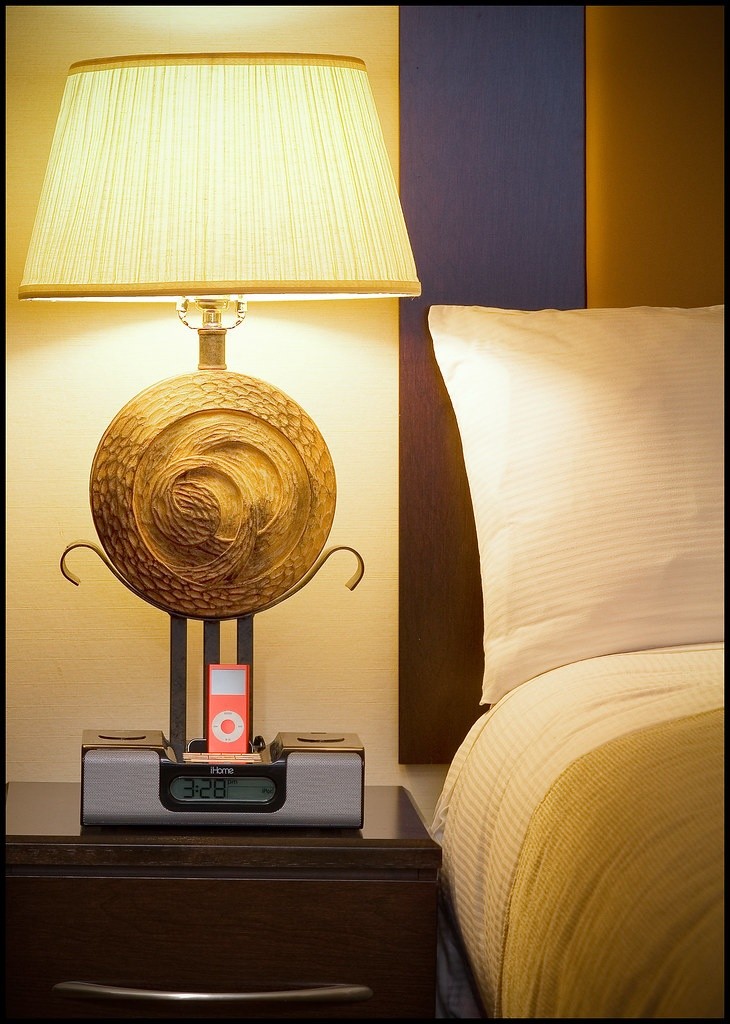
429;302;725;706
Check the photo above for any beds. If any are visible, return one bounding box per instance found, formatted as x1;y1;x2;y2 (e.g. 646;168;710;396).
396;6;725;1019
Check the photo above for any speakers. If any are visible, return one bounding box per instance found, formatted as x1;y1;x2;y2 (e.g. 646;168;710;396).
81;730;365;829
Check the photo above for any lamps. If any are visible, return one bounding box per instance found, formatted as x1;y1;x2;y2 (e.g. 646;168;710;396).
18;52;421;753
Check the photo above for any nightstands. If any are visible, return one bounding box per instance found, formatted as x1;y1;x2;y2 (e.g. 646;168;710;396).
5;782;443;1019
